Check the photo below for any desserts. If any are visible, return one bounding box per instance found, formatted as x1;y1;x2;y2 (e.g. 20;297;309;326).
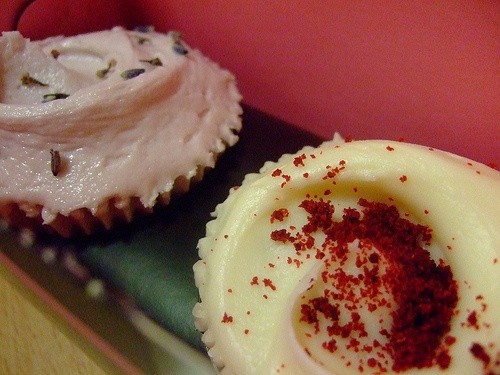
190;135;500;375
0;22;245;239
